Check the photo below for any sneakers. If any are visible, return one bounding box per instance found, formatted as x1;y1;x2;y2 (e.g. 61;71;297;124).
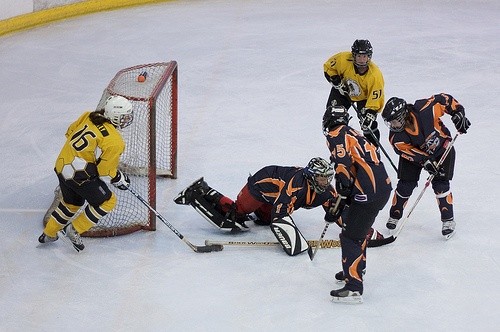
440;219;456;239
173;177;209;205
386;218;399;235
57;223;84;252
36;232;59;248
335;268;367;285
329;285;364;304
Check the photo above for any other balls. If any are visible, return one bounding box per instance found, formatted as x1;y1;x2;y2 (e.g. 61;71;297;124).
307;223;329;260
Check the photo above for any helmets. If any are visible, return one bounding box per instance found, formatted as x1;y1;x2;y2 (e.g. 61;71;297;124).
351;39;373;67
105;96;134;127
381;97;406;132
303;157;334;194
322;105;349;135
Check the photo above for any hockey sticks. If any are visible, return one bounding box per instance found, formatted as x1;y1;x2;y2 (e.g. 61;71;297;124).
345;93;420;188
123;182;224;253
204;240;341;248
366;132;460;248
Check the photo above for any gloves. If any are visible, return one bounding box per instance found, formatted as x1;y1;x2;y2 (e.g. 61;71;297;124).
423;158;445;177
366;227;384;240
324;194;347;222
329;75;350;96
451;110;471;133
359;108;378;131
109;170;131;189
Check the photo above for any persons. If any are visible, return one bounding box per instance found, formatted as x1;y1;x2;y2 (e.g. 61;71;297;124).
321;104;393;304
323;39;384;159
380;93;470;240
173;157;384;242
37;96;134;252
